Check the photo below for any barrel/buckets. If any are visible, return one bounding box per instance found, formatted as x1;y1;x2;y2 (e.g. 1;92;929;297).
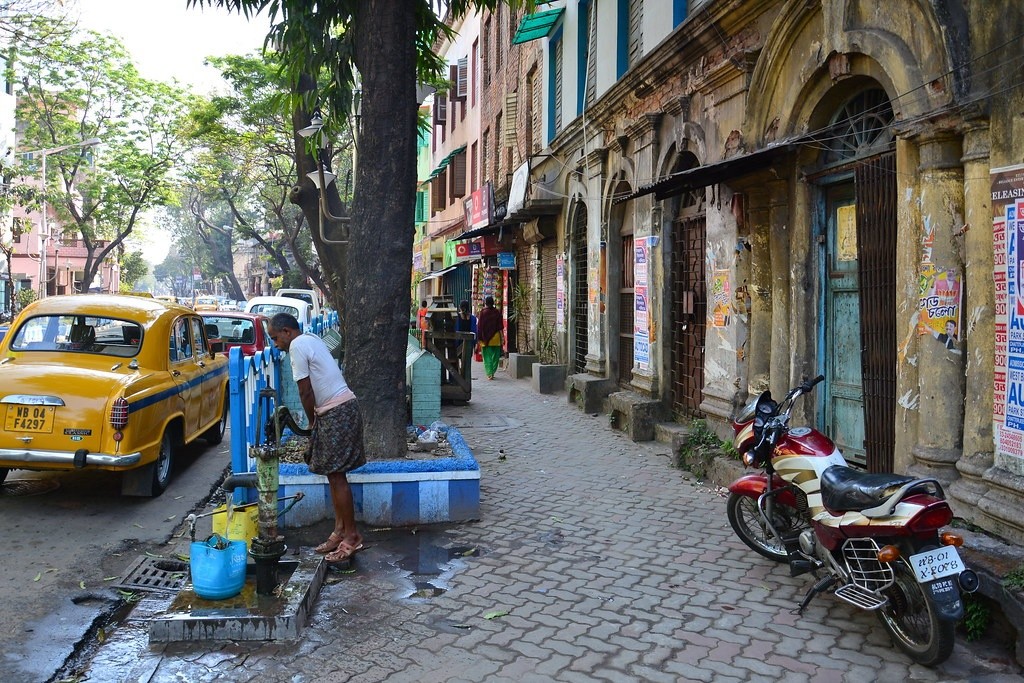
212;503;259;551
189;534;248;601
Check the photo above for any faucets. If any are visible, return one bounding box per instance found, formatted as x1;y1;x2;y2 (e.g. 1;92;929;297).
217;471;258;493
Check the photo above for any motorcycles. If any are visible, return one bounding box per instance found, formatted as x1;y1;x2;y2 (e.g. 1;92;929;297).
726;374;982;668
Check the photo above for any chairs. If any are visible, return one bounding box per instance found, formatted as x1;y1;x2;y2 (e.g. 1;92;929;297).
205;323;219;342
69;325;96;351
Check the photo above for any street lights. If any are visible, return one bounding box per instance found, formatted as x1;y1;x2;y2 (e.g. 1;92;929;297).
39;137;102;298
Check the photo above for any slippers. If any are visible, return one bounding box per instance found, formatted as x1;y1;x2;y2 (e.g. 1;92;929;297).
315;532;344;552
325;541;363;562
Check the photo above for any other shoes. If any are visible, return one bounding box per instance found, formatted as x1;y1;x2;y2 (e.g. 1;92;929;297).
486;374;495;380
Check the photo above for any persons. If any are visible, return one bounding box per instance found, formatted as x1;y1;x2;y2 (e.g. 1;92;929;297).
415;301;429;349
925;320;957;349
267;312;367;563
477;297;505;379
455;297;477;380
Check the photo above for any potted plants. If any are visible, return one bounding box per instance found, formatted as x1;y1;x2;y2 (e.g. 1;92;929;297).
508;283;539;377
531;308;565;395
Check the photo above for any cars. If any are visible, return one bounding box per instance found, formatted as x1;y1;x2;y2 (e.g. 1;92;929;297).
0;294;230;501
156;287;323;362
